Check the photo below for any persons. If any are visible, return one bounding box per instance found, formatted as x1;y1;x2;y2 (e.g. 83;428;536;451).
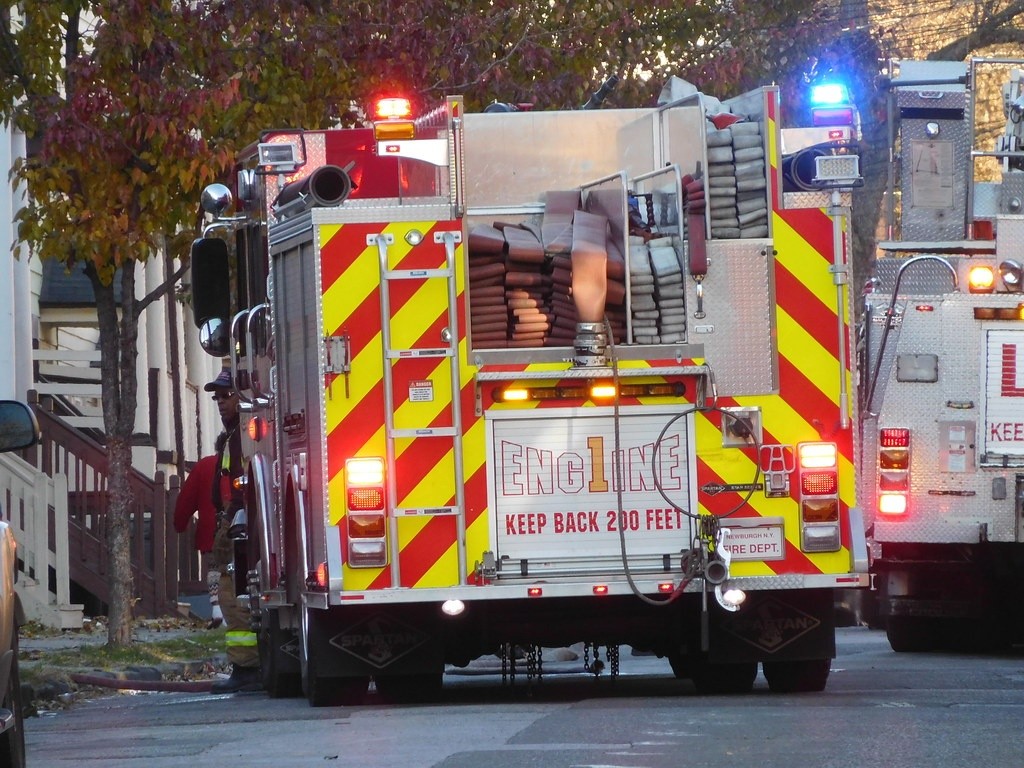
174;432;235;626
204;370;264;695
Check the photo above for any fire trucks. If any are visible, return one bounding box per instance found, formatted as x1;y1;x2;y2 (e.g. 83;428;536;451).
864;55;1024;653
188;74;875;701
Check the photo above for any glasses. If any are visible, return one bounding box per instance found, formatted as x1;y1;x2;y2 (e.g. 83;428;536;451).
212;391;236;401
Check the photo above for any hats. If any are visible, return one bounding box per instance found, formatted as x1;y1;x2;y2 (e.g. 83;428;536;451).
204;369;232;392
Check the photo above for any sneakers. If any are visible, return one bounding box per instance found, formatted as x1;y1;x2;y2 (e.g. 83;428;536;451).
210;665;261;694
